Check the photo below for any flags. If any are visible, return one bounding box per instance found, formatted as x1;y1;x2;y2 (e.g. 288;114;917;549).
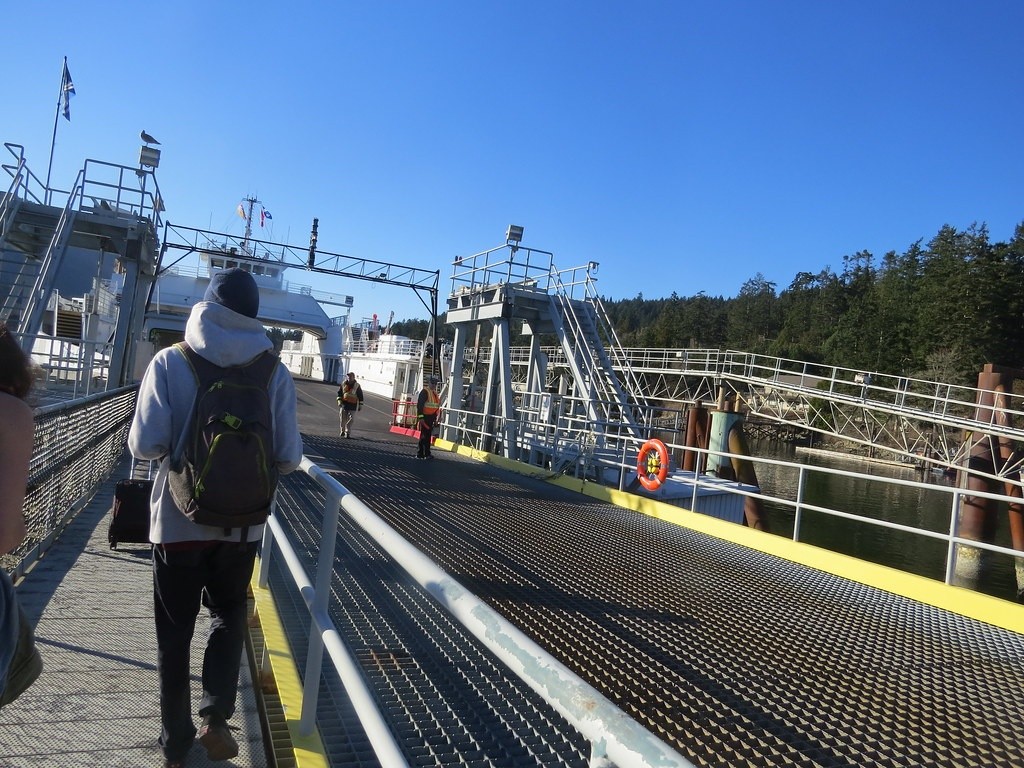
60;62;76;123
236;204;273;227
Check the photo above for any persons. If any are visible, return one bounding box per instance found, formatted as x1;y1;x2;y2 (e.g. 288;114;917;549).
0;320;44;710
124;265;302;768
336;372;364;439
417;377;441;459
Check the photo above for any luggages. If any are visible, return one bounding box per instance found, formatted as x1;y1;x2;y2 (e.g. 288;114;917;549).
109;459;155;551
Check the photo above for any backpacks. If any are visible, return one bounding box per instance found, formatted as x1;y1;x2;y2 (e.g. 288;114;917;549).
170;342;281;528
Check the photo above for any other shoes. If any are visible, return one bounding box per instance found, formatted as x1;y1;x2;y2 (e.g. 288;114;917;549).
424;453;431;458
416;455;421;458
340;431;343;436
198;715;242;762
344;434;350;437
160;755;185;768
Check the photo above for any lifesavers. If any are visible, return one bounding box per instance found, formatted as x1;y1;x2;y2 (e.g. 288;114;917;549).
634;438;669;493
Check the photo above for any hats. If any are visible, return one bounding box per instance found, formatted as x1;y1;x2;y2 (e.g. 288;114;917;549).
430;378;439;383
347;372;354;376
204;268;260;318
